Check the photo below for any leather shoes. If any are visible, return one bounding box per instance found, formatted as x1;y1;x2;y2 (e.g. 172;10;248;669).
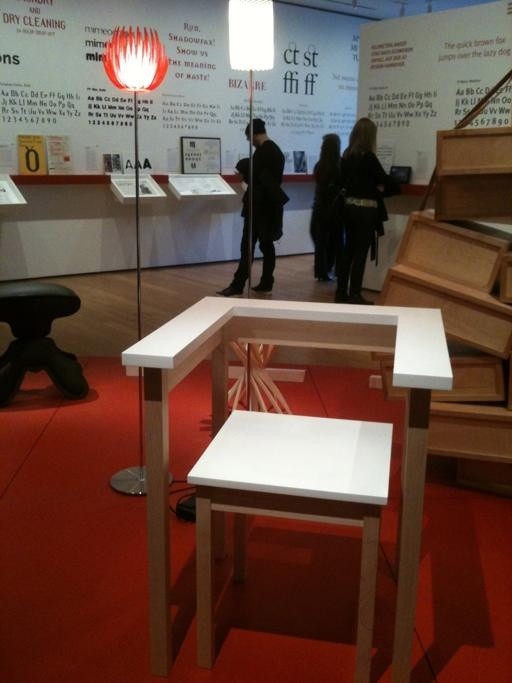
317;272;336;282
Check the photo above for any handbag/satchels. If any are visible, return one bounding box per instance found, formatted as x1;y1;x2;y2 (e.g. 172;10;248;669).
328;168;388;221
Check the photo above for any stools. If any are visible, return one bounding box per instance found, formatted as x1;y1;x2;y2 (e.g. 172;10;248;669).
0;280;88;411
187;408;395;682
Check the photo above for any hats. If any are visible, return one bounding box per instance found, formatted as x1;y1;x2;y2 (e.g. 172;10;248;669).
244;119;267;135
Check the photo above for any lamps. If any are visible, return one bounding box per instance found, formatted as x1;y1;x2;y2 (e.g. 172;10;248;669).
229;1;276;408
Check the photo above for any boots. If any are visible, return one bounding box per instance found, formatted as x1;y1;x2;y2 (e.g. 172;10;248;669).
333;271;374;305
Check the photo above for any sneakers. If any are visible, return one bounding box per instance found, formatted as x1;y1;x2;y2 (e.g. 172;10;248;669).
251;282;272;291
215;285;244;298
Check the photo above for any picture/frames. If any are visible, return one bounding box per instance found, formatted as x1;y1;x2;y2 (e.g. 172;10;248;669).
180;136;222;175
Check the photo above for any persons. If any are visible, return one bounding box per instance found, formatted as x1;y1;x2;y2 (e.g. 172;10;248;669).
309;133;343;282
338;117;398;304
217;117;289;294
